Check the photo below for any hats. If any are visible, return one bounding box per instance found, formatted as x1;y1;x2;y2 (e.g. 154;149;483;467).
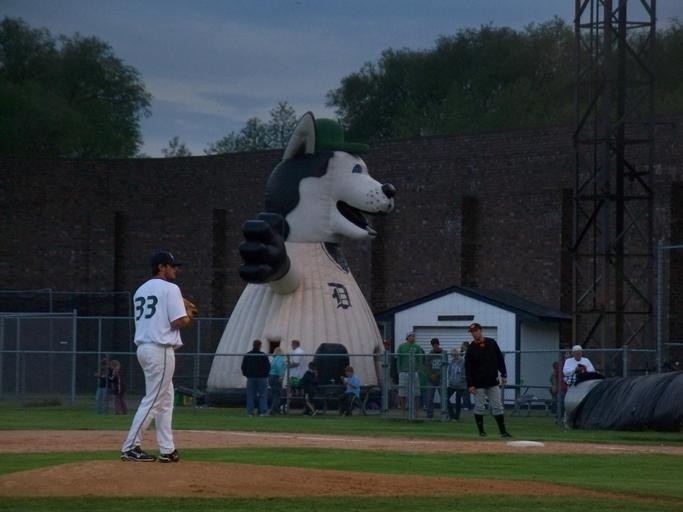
466;323;481;332
403;331;415;339
151;250;184;268
429;338;440;345
571;344;583;353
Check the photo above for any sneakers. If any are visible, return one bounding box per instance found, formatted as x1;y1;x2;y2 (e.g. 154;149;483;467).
257;412;271;418
500;433;514;442
311;409;319;417
119;445;156;464
158;449;181;464
300;412;309;417
247;412;254;418
477;433;488;441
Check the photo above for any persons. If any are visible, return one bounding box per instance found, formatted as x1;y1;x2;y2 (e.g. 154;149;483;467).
267;346;287;415
550;343;596;429
119;249;194;464
335;365;361;417
240;339;271;417
300;362;320;416
281;338;308;413
93;358;112;416
109;359;129;416
383;321;513;440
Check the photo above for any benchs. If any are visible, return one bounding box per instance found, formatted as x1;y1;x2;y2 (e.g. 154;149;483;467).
280;386;376;416
518;399;553;416
